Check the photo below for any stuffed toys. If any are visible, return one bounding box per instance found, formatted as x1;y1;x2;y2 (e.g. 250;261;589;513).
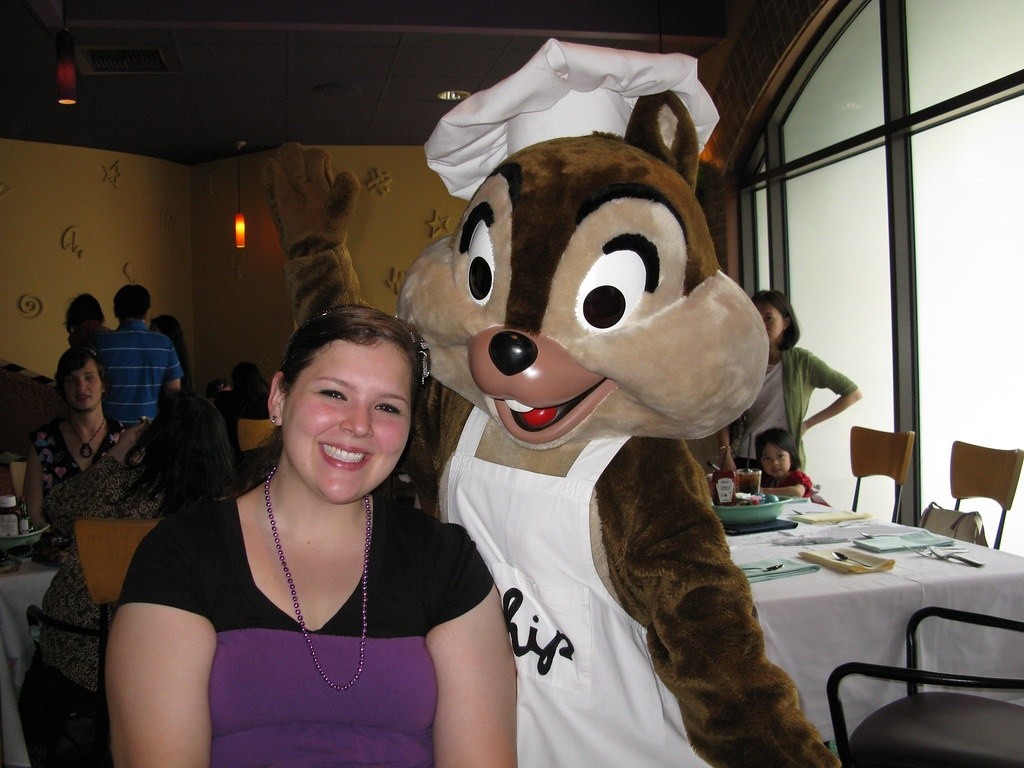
273;38;840;768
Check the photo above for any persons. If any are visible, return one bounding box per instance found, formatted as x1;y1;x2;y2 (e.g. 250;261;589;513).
102;305;519;768
21;347;124;551
205;361;270;461
65;294;112;348
89;285;184;428
718;290;864;488
757;429;813;497
17;396;233;768
150;314;204;399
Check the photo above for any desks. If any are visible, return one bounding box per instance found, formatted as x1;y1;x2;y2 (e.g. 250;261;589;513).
0;558;60;768
723;500;1024;749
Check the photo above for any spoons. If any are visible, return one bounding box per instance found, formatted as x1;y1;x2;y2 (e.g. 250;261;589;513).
792;509;830;515
860;532;898;537
742;564;783;572
832;552;872;567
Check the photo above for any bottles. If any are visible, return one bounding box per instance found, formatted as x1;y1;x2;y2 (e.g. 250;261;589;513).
0;495;19;536
712;470;738;506
18;496;34;534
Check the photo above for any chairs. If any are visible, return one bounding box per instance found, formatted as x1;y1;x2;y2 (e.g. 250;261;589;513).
950;441;1024;550
9;419;278;768
826;606;1024;768
851;427;915;523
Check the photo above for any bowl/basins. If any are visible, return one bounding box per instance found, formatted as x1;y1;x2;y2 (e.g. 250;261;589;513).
713;495;793;522
0;523;50;551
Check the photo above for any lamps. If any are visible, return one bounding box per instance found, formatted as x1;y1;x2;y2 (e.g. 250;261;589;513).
234;140;246;248
54;0;76;105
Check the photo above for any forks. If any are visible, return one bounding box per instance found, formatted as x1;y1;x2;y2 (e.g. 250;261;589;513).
927;545;983;567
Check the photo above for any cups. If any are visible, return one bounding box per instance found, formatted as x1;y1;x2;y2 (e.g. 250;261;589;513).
736;468;762;495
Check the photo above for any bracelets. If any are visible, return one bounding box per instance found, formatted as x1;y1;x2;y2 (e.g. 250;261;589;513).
720;446;730;449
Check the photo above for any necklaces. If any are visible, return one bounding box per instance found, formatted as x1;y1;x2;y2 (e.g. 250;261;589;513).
67;417;105;458
266;468;371;690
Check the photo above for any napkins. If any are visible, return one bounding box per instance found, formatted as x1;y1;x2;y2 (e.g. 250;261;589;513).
786;512;872;525
853;530;956;554
800;548;894;574
737;558;819;584
